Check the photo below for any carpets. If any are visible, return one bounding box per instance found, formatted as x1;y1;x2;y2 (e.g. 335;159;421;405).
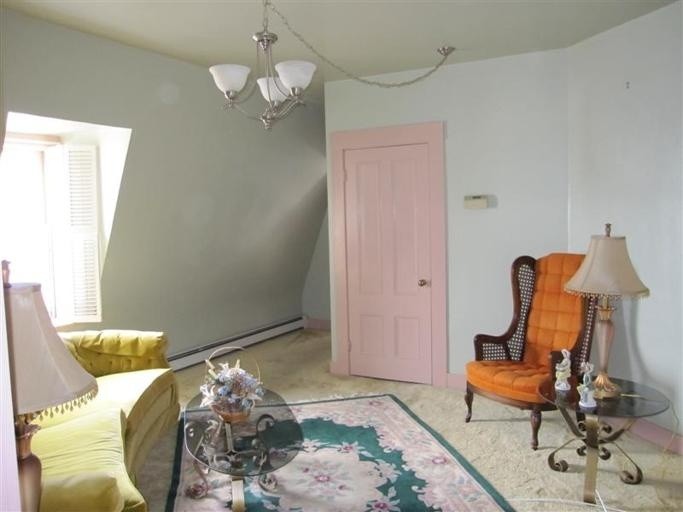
165;395;518;512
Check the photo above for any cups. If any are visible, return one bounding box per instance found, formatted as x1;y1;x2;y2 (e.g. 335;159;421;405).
577;386;595;404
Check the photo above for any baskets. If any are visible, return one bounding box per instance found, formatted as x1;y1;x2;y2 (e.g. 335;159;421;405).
202;345;261;423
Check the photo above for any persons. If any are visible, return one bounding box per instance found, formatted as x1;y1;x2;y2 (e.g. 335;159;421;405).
555;349;572;390
577;361;596;407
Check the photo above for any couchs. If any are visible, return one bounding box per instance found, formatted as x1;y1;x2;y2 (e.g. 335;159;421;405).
18;330;181;512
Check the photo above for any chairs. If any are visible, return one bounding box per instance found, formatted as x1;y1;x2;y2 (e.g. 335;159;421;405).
464;253;597;450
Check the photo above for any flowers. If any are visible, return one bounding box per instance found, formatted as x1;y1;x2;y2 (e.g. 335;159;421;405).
199;359;265;413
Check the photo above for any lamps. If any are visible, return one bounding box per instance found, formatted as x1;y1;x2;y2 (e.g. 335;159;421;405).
564;224;650;399
3;262;98;512
209;0;317;131
